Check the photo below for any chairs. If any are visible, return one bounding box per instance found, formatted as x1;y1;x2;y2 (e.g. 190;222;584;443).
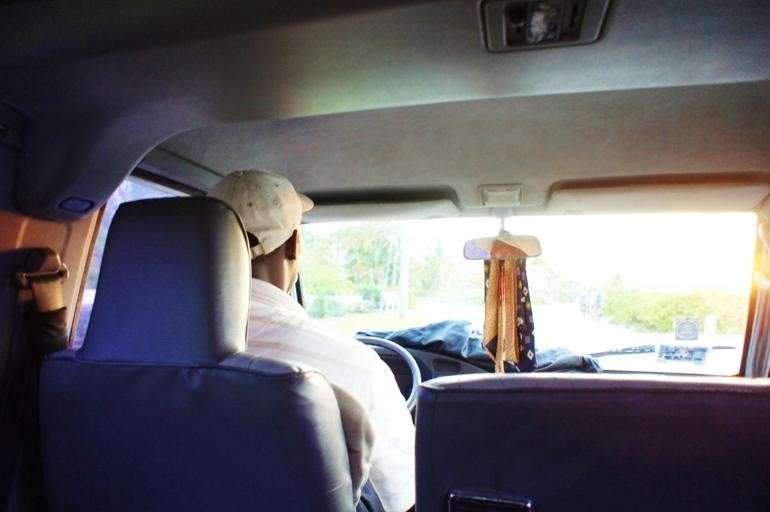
415;372;769;510
37;194;354;507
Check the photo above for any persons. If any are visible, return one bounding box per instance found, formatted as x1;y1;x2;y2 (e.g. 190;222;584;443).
199;167;421;511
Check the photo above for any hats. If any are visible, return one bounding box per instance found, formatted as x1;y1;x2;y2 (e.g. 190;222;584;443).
206;169;315;260
